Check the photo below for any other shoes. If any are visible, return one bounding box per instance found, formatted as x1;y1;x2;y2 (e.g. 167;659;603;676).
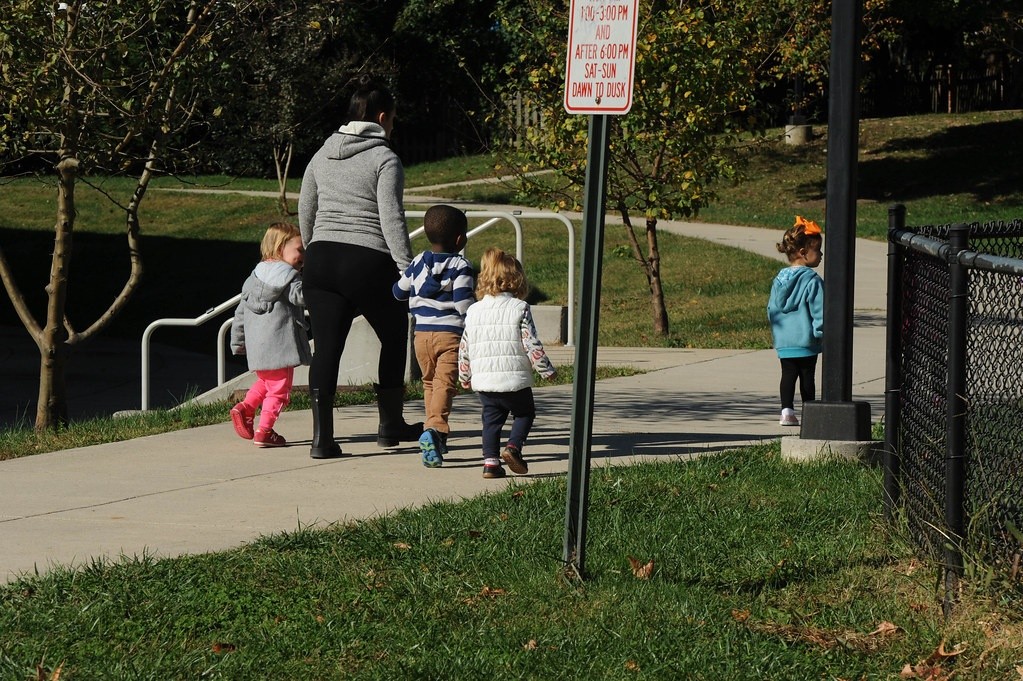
779;414;799;425
436;430;449;454
482;460;506;478
418;428;443;468
501;446;528;474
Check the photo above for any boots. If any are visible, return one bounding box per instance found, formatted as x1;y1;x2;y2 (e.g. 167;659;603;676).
309;388;342;458
373;383;425;447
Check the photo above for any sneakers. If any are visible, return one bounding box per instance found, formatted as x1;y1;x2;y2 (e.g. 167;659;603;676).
253;428;286;447
230;401;255;440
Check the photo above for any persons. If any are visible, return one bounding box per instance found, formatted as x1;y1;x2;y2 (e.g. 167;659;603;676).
230;222;313;448
392;205;475;470
767;215;824;426
298;81;424;459
458;247;559;479
